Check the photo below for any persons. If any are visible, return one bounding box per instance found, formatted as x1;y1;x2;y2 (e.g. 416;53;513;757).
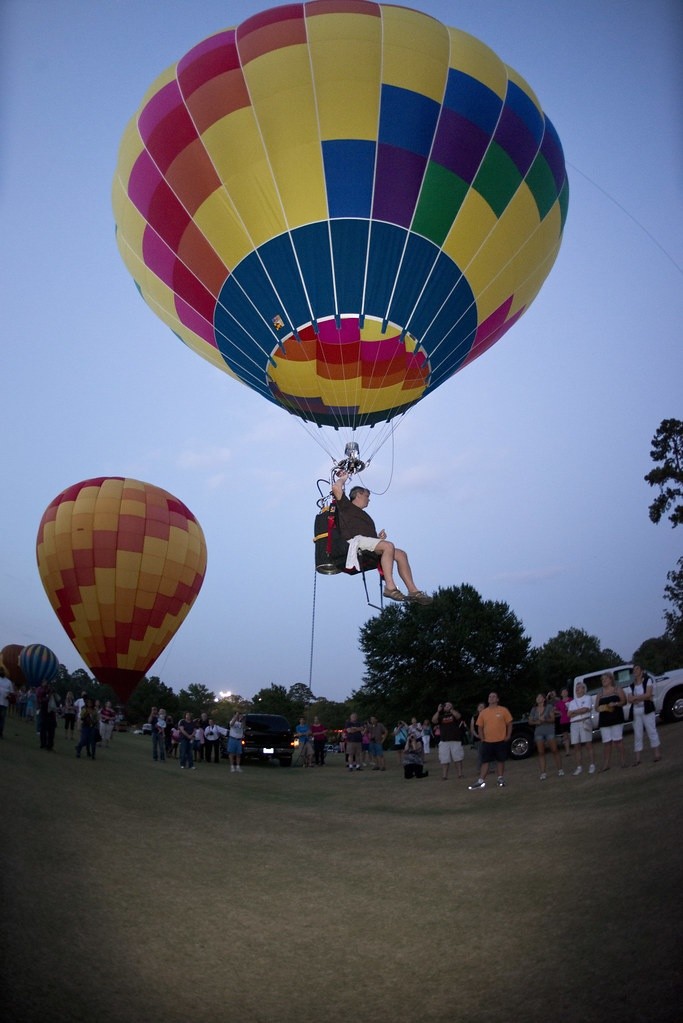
332;471;433;606
0;666;488;774
567;682;596;776
596;672;630;769
468;691;513;791
431;701;464;780
627;665;661;767
527;692;565;780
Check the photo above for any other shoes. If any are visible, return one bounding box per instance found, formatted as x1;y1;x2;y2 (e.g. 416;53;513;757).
632;761;640;767
236;768;242;773
181;766;184;769
380;766;385;771
230;768;235;772
588;764;595;774
191;767;197;770
540;772;547;780
348;768;353;772
653;755;662;762
372;767;379;770
598;767;609;773
572;766;582;776
468;779;485;789
558;768;564;776
356;767;363;771
496;776;505;786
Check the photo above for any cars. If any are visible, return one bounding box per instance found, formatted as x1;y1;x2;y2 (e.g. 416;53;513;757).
508;714;571;759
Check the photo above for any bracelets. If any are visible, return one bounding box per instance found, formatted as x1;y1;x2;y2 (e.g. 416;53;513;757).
449;707;453;713
616;702;618;706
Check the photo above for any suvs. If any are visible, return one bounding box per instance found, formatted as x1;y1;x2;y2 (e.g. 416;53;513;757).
220;713;294;766
571;666;682;734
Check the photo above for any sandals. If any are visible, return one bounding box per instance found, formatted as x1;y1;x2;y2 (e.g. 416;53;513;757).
383;585;408;601
408;590;433;605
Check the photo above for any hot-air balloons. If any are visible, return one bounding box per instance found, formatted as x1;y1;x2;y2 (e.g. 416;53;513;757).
0;644;28;694
30;478;206;706
113;5;573;476
16;642;57;697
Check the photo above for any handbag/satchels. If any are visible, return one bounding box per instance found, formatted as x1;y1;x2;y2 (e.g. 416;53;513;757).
583;721;592;732
